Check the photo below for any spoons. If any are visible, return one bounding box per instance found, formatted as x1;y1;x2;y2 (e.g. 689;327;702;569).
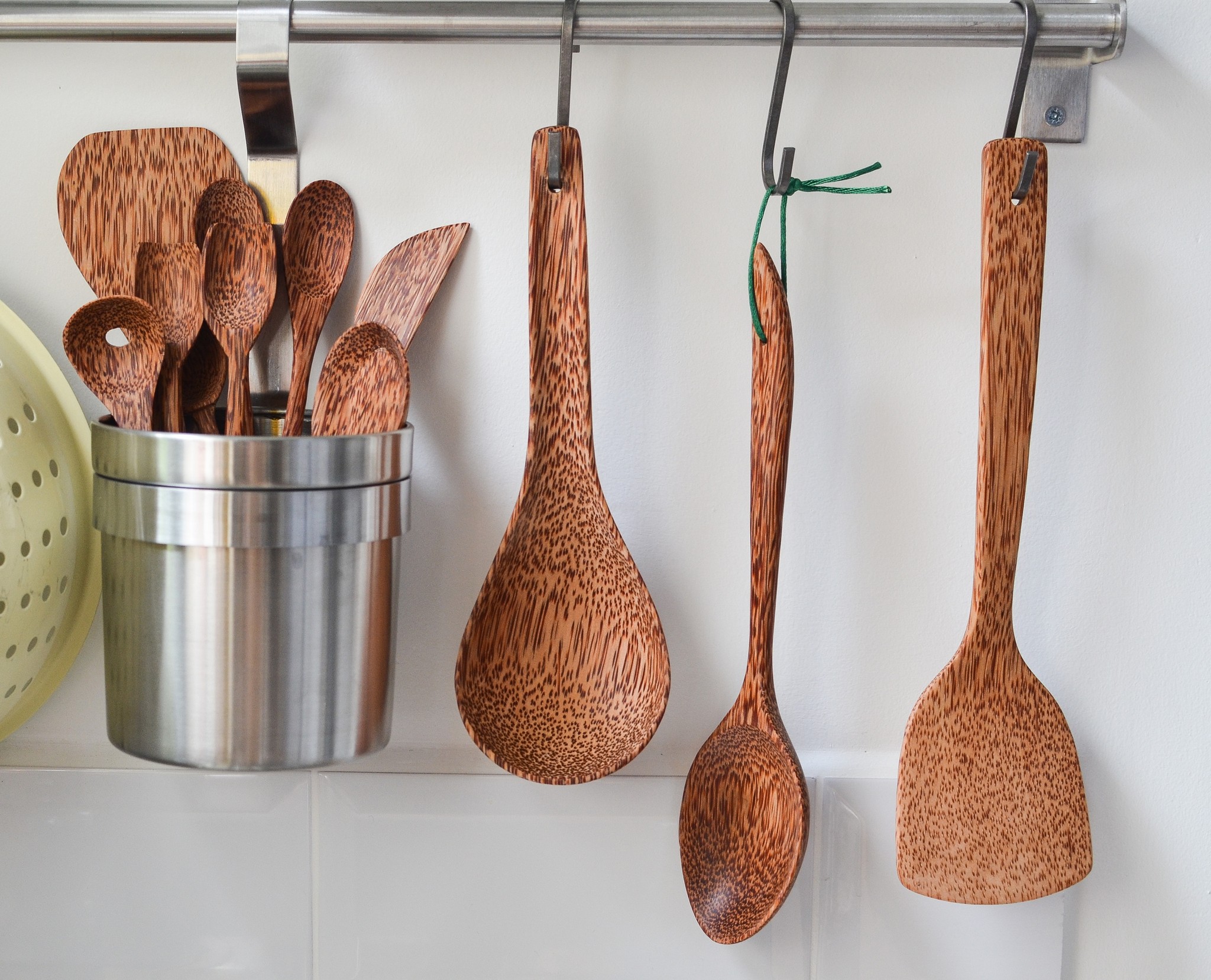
61;294;166;437
312;322;410;436
191;177;264;436
677;244;813;950
132;242;203;432
198;222;278;437
283;180;355;437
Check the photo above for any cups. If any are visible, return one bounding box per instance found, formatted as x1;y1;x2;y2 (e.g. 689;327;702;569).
89;407;414;772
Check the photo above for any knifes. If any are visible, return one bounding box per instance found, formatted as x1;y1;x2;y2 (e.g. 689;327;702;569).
357;222;471;354
